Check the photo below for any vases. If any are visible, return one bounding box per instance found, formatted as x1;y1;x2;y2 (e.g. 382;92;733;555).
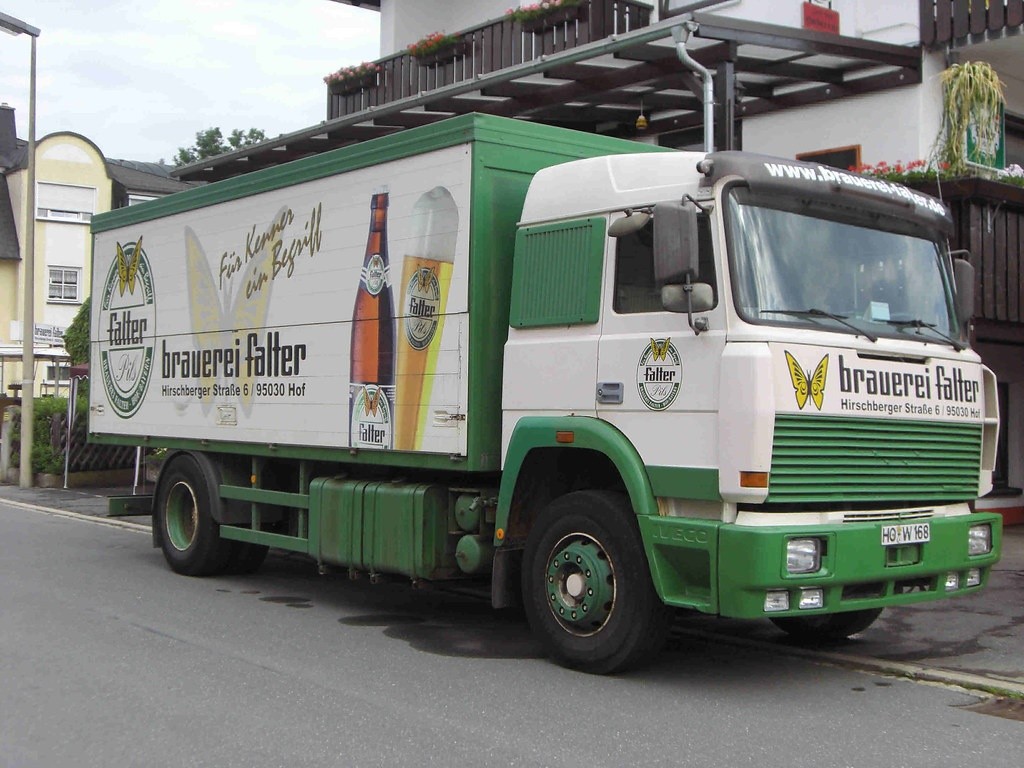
521;7;586;35
327;75;374;96
418;43;469;66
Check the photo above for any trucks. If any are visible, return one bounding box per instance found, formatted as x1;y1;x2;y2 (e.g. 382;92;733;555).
84;112;1005;678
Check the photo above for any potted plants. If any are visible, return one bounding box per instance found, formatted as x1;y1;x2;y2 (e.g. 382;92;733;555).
31;444;65;488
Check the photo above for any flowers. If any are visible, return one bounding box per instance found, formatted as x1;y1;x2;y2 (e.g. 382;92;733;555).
406;32;466;58
513;0;581;20
323;62;382;84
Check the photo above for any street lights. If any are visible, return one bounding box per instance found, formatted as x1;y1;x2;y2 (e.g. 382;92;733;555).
0;8;38;486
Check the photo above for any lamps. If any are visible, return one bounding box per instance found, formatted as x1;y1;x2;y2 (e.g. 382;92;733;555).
634;99;649;128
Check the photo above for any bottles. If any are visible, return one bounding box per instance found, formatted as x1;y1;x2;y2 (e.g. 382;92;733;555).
348;166;397;450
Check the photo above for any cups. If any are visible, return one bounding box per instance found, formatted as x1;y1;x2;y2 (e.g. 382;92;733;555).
392;185;460;451
